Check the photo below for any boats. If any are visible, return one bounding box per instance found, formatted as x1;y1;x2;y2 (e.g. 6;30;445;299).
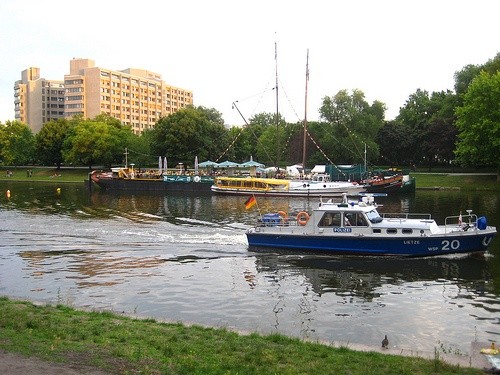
210;174;369;198
88;162;417;196
244;191;499;258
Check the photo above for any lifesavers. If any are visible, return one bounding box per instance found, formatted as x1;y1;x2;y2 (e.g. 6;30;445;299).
278;211;286;223
194;176;199;183
297;212;309;225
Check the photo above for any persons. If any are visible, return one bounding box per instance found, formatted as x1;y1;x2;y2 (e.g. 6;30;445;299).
5;168;33;180
129;165;333;191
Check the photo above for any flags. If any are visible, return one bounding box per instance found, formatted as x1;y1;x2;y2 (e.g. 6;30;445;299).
458;213;463;227
244;195;257;210
